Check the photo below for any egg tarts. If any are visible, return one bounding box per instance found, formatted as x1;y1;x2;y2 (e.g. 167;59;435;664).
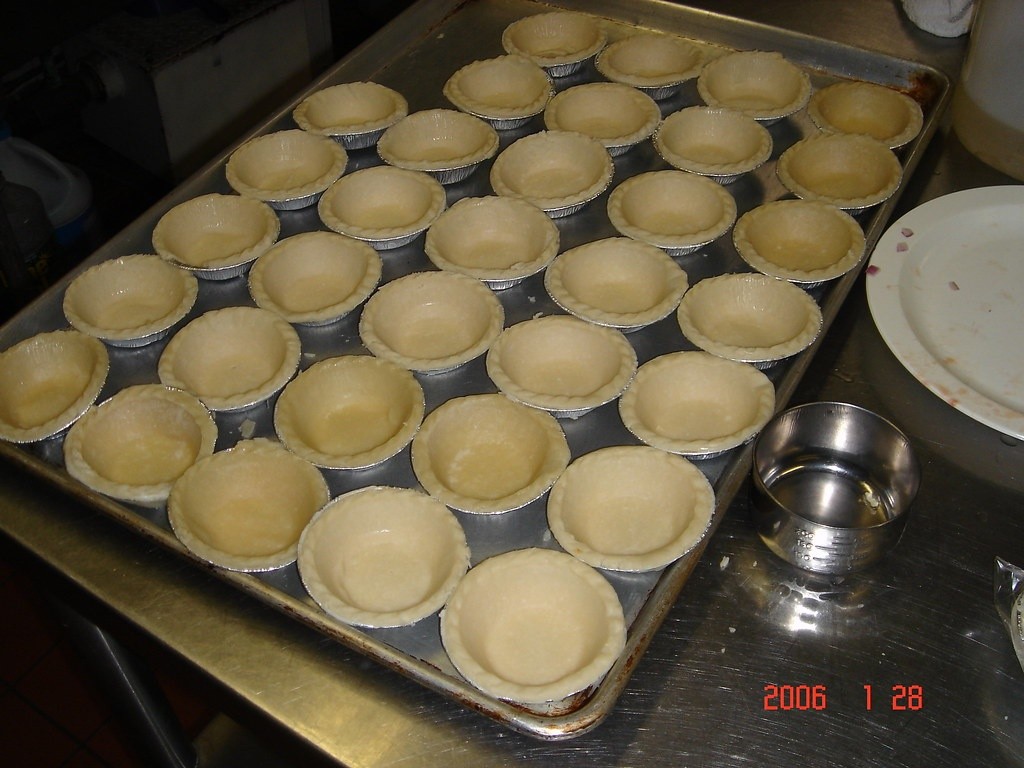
0;10;924;711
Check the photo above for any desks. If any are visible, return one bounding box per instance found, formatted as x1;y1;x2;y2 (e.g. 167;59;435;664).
0;0;1024;768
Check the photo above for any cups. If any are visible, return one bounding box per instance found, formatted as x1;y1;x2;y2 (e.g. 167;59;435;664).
752;402;921;574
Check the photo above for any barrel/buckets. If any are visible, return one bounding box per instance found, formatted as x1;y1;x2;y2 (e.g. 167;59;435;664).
951;0;1024;183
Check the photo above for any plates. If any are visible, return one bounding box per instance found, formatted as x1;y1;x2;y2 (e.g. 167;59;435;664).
865;184;1024;442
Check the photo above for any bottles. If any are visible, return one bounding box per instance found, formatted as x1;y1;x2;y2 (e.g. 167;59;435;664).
0;123;105;323
